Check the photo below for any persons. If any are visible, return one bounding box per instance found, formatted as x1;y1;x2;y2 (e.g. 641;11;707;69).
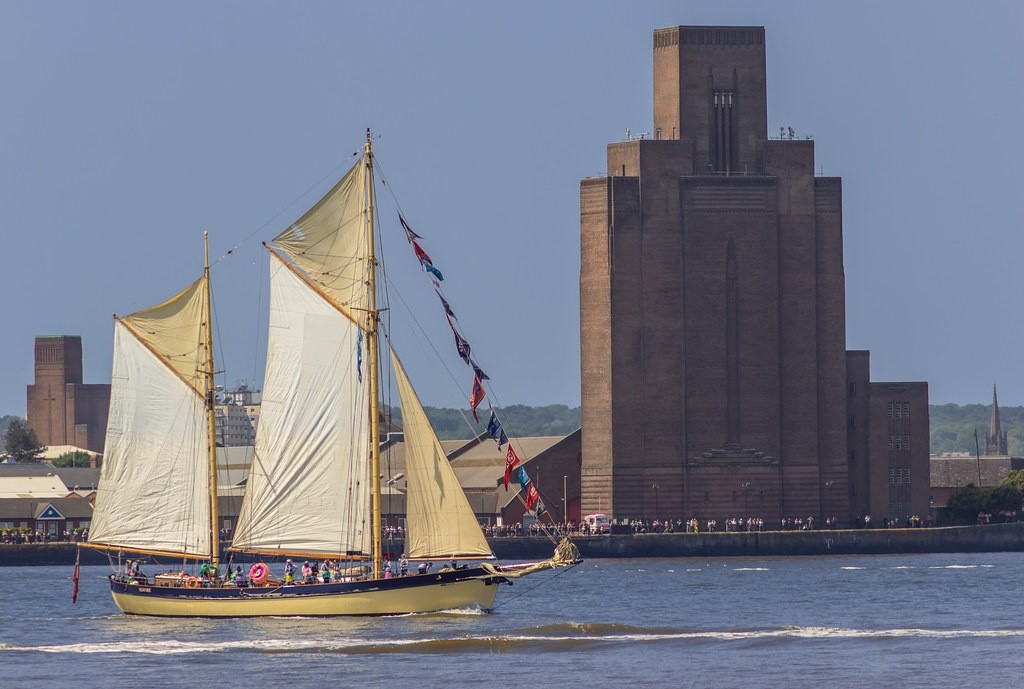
124;554;458;587
220;528;233;541
3;527;88;544
481;507;1024;537
383;526;407;536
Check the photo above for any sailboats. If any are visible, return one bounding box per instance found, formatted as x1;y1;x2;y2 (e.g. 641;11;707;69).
72;125;585;616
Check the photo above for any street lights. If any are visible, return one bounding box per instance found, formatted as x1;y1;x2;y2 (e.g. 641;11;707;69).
741;482;751;532
826;481;835;531
652;483;661;535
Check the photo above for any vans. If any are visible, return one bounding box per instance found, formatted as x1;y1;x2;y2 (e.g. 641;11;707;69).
585;513;610;535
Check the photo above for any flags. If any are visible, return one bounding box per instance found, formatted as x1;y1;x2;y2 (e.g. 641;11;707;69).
397;211;549;523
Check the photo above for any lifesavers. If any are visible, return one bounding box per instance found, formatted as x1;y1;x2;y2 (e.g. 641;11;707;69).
185;575;200;590
248;563;270;584
